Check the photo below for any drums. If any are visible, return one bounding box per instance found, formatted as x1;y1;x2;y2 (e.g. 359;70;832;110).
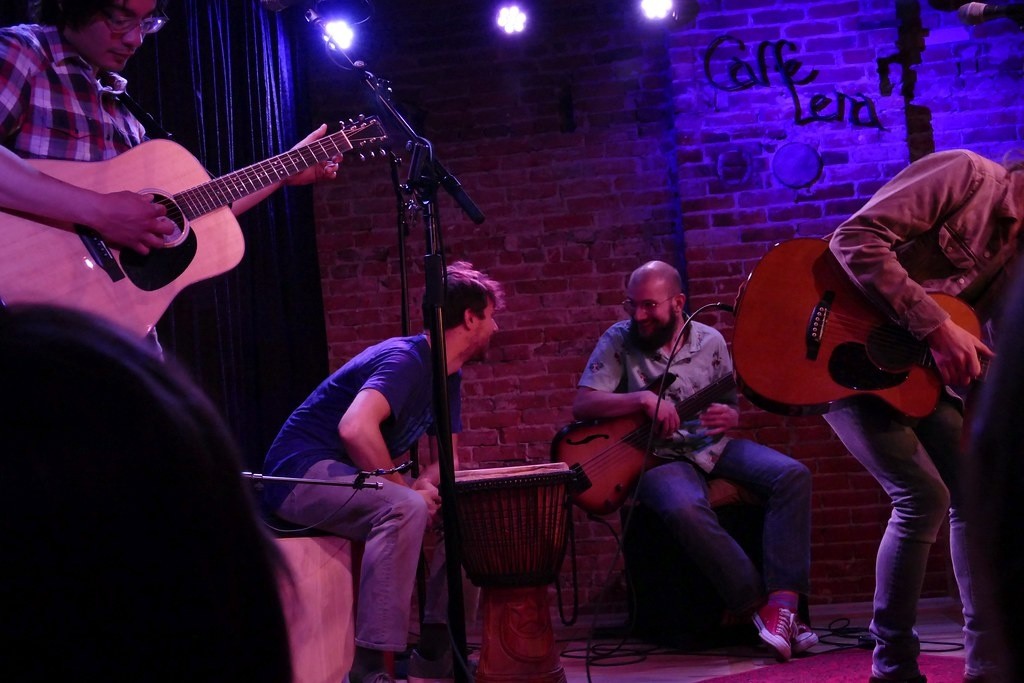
453;462;578;683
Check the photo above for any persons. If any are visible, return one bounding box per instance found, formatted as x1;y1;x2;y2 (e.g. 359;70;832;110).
261;261;499;683
0;0;344;361
822;149;1024;683
572;260;819;660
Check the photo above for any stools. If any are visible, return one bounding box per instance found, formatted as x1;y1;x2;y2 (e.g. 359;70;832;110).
270;536;354;683
619;480;810;650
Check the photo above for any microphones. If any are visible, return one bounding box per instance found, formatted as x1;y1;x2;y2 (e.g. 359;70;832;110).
260;0;327;13
959;1;1024;26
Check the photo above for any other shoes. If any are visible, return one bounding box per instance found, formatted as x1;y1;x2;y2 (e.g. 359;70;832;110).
868;673;927;683
407;642;478;683
349;667;397;683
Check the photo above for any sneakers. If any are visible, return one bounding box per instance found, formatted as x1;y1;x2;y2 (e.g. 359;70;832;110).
751;600;797;659
792;620;819;653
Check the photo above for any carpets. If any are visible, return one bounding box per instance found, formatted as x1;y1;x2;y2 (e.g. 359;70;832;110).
694;646;966;683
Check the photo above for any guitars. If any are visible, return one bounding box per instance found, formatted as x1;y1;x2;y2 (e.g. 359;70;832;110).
549;370;736;518
2;109;392;341
731;235;995;429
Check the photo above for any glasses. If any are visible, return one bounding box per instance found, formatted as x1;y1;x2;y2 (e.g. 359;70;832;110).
98;8;170;34
621;295;677;316
